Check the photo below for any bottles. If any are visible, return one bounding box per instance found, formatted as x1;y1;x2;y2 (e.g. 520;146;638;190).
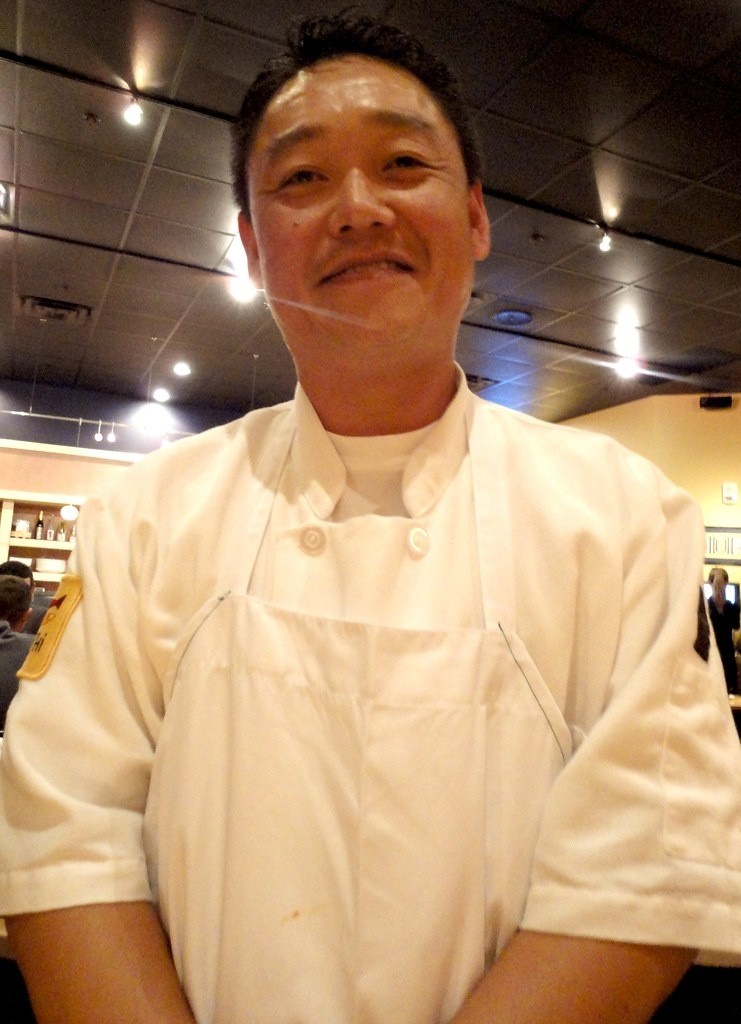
56;519;65;541
69;524;76;543
47;514;54;540
35;510;43;540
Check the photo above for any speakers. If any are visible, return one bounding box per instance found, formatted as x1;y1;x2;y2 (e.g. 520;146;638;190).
700;396;733;409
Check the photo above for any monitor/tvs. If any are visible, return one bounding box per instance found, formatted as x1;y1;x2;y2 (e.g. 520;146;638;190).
702;582;739;606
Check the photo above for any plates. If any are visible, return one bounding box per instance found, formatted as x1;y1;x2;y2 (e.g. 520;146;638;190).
9;556;32;567
36;558;66;574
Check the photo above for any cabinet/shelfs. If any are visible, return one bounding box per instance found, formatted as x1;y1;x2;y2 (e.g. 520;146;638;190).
1;491;85;581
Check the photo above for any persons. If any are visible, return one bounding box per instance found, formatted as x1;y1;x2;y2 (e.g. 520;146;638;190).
0;561;53;738
0;11;741;1024
706;568;741;695
